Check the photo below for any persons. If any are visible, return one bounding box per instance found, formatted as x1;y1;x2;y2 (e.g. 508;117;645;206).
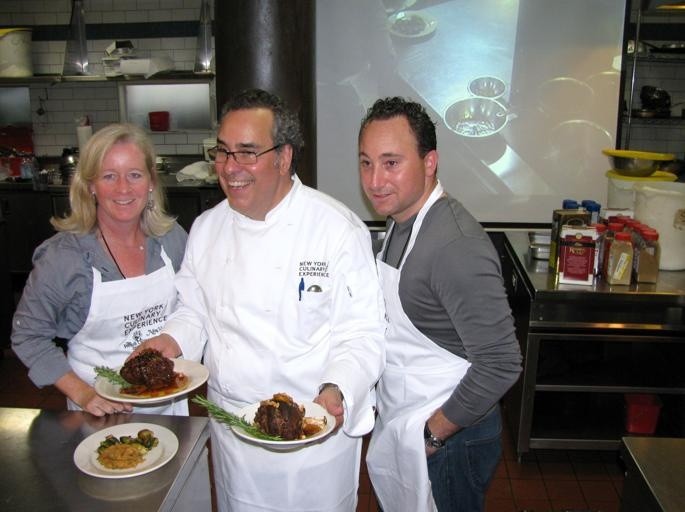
10;122;191;416
358;95;523;512
123;88;387;512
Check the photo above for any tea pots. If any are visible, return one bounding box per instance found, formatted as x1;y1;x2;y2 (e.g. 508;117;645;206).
59;146;79;170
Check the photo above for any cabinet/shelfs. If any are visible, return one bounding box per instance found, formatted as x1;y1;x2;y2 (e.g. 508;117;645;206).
506;238;685;467
617;0;684;176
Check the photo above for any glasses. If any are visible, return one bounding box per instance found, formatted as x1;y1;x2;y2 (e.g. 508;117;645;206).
207;144;286;164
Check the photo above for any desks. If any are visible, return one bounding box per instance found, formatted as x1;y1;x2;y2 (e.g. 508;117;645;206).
618;435;684;509
0;406;213;512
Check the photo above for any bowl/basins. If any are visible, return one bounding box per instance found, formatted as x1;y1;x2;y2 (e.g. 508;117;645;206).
442;95;509;142
466;74;508;100
532;70;620;187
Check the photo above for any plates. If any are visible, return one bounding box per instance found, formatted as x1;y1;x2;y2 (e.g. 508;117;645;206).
73;421;180;480
229;396;336;451
93;354;210;405
78;464;178;502
386;9;438;39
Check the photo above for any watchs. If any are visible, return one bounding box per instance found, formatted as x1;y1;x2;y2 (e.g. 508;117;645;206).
424;420;445;450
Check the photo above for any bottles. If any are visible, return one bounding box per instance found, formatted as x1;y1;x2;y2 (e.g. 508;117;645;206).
560;196;661;286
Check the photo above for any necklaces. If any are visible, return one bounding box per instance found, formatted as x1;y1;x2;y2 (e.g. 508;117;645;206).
384;225;413;267
99;228;128;280
